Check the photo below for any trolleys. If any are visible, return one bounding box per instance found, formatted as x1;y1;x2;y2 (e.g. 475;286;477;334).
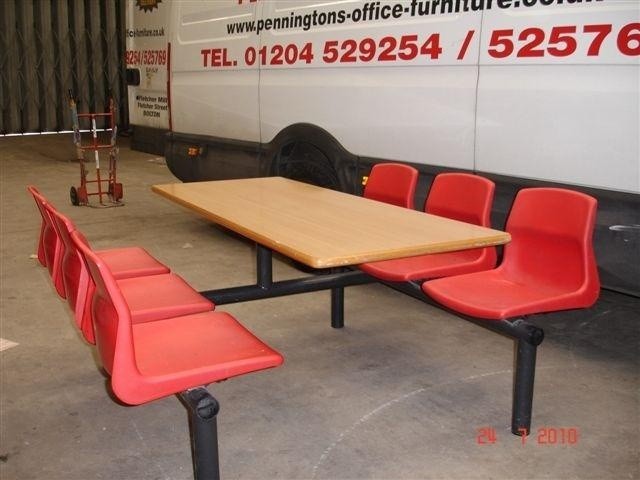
68;86;125;210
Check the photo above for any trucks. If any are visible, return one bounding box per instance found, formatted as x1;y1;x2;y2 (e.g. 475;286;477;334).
124;0;640;299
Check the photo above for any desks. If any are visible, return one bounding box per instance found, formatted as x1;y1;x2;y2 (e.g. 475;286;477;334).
149;172;513;328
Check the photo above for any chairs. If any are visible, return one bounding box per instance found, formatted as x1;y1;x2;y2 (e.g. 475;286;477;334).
422;186;601;437
345;173;499;296
68;230;285;480
363;162;417;206
27;184;217;344
